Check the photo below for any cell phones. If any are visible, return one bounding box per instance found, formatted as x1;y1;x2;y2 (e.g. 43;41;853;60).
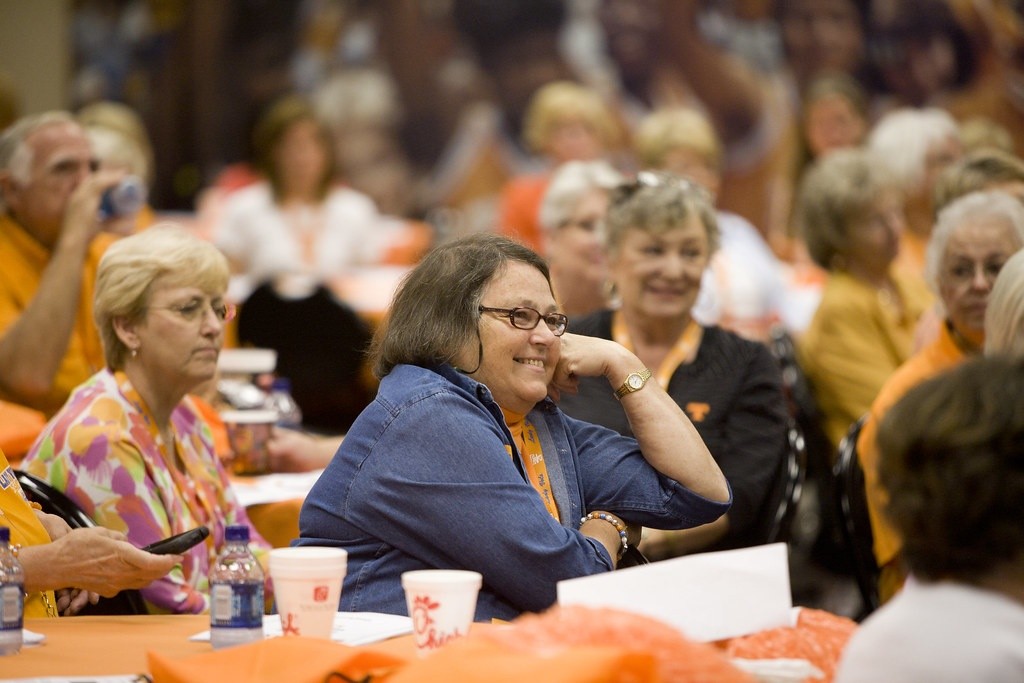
137;526;209;557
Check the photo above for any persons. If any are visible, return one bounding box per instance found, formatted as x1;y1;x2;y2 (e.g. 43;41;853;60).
19;224;282;615
557;169;790;572
1;449;184;617
295;236;735;623
840;356;1024;681
0;1;1024;682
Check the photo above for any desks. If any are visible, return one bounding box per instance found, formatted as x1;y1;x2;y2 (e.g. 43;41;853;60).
0;392;392;683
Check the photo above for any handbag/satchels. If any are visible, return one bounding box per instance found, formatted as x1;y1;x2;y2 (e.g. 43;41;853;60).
744;331;880;633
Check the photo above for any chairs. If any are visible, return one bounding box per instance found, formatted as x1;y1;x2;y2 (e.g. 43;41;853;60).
745;331;881;620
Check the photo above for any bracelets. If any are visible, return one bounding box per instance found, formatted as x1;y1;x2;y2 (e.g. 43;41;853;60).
578;512;627;561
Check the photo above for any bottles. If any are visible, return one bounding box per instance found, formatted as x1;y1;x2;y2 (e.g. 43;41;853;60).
0;527;24;655
262;378;302;432
209;525;264;648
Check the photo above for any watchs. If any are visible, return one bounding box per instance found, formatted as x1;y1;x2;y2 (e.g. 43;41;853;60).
613;367;652;400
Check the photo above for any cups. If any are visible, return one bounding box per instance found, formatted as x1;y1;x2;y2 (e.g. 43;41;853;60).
220;411;277;475
401;570;483;658
269;547;348;641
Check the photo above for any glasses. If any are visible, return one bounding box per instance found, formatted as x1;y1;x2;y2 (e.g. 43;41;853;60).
614;171;703;192
144;301;240;323
476;304;568;337
